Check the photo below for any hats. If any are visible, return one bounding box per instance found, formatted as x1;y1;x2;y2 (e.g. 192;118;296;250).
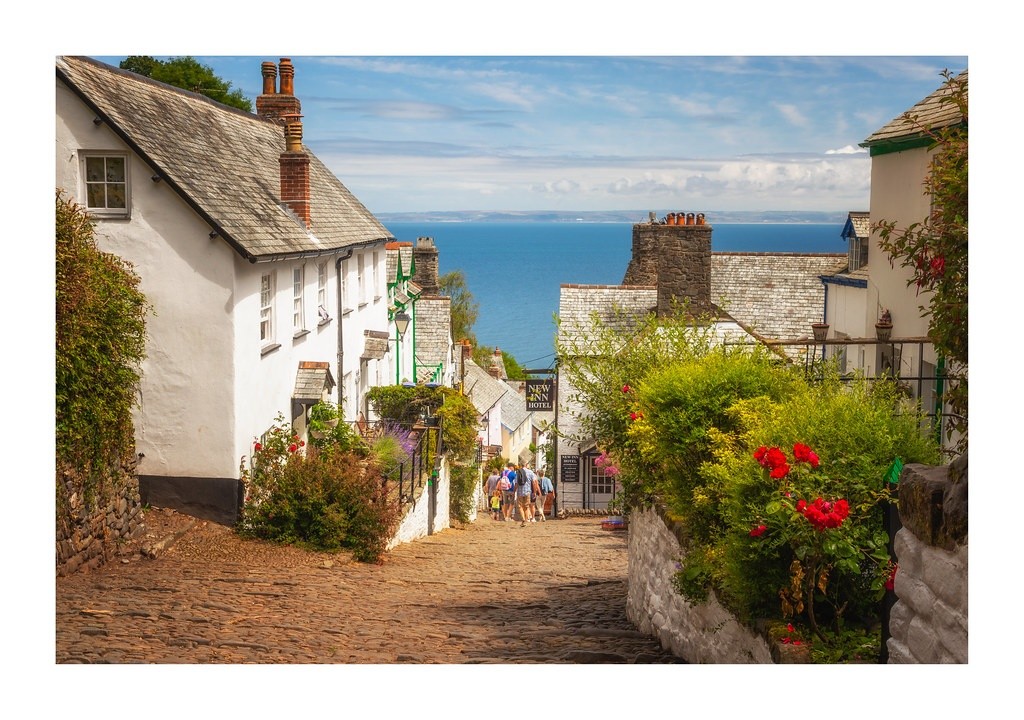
508;463;516;466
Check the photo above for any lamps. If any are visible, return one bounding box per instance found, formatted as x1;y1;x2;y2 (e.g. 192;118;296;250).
388;309;412;342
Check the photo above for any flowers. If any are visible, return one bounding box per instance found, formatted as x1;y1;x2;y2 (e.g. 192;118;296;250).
875;303;889;324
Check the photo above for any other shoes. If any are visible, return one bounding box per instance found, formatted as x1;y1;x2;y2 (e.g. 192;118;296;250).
521;521;526;527
531;518;535;522
543;516;545;522
505;516;515;521
527;519;530;523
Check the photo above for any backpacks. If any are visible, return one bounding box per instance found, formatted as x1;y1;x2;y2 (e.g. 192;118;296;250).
499;470;512;490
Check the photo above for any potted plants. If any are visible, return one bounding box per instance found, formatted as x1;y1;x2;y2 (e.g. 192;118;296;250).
309;401;339;439
608;499;623;521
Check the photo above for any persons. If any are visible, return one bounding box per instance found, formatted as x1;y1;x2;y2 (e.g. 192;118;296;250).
484;459;555;527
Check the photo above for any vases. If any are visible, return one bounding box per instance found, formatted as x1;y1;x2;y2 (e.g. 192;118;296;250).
875;323;893;340
813;324;829;341
601;520;623;528
